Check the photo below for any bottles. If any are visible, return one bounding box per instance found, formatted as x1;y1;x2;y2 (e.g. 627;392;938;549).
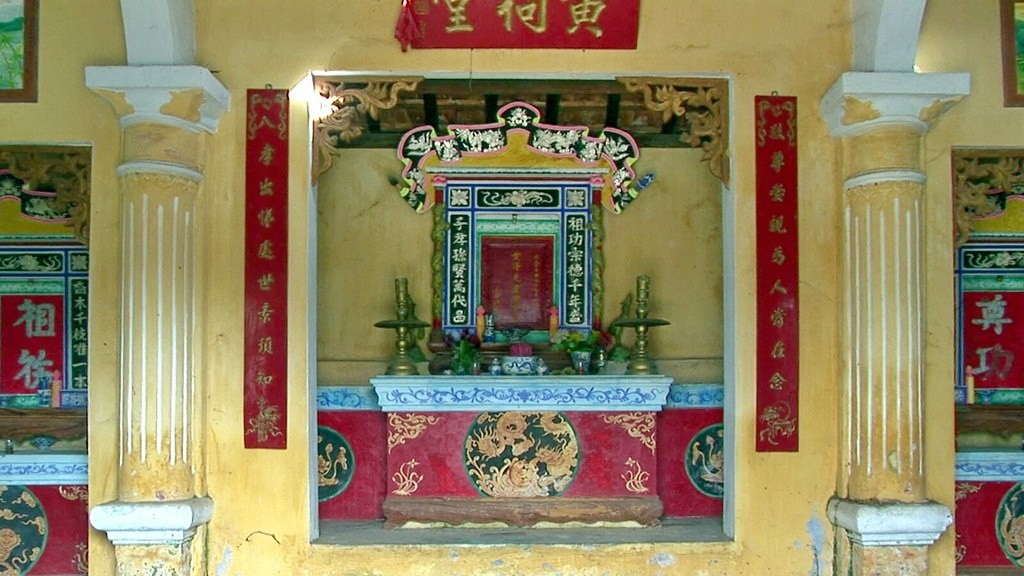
6;439;13;453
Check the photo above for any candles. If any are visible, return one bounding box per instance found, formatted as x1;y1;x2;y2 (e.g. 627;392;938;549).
965;366;974;407
51;369;62;409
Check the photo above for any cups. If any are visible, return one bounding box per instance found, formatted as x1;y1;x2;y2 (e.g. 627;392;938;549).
37;390;51;406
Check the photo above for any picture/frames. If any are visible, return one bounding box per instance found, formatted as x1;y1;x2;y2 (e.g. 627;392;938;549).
999;0;1024;108
0;0;38;103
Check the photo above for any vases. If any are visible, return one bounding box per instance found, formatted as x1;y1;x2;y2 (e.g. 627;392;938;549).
573;351;590;375
451;352;479;375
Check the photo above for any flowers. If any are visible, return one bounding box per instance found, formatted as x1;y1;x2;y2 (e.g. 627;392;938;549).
550;318;610;355
444;325;480;356
